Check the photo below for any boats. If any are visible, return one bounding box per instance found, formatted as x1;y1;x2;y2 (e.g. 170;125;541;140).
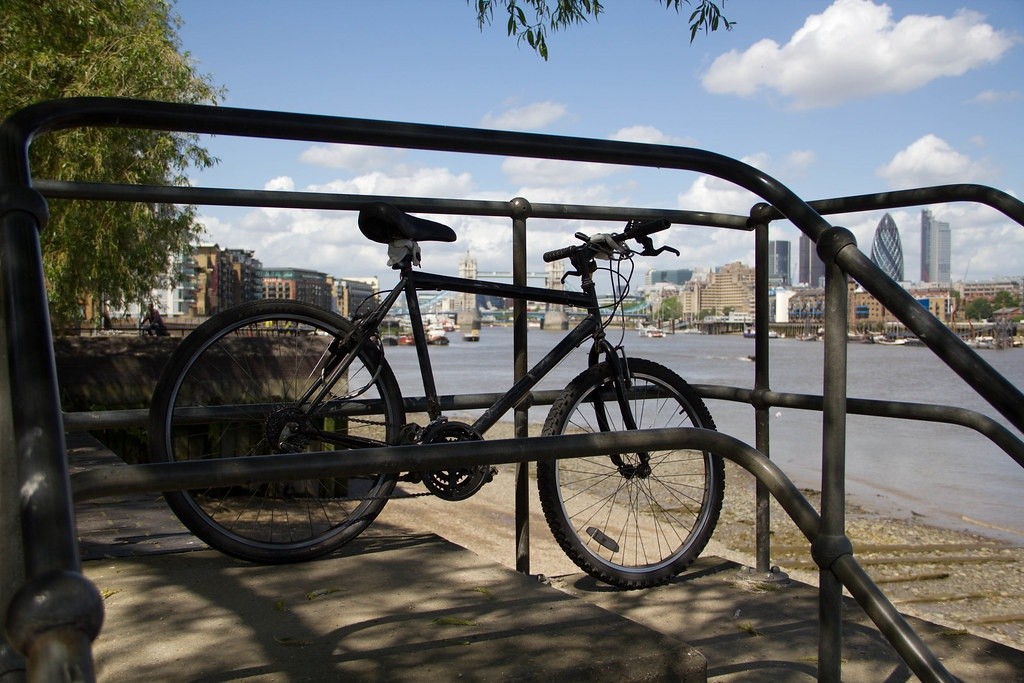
462;328;481;342
639;324;667;338
741;318;1024;350
374;308;462;346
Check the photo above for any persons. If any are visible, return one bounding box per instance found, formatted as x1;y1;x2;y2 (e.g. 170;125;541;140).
140;304;164;337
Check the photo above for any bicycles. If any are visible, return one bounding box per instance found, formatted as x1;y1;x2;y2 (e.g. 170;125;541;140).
147;201;727;588
